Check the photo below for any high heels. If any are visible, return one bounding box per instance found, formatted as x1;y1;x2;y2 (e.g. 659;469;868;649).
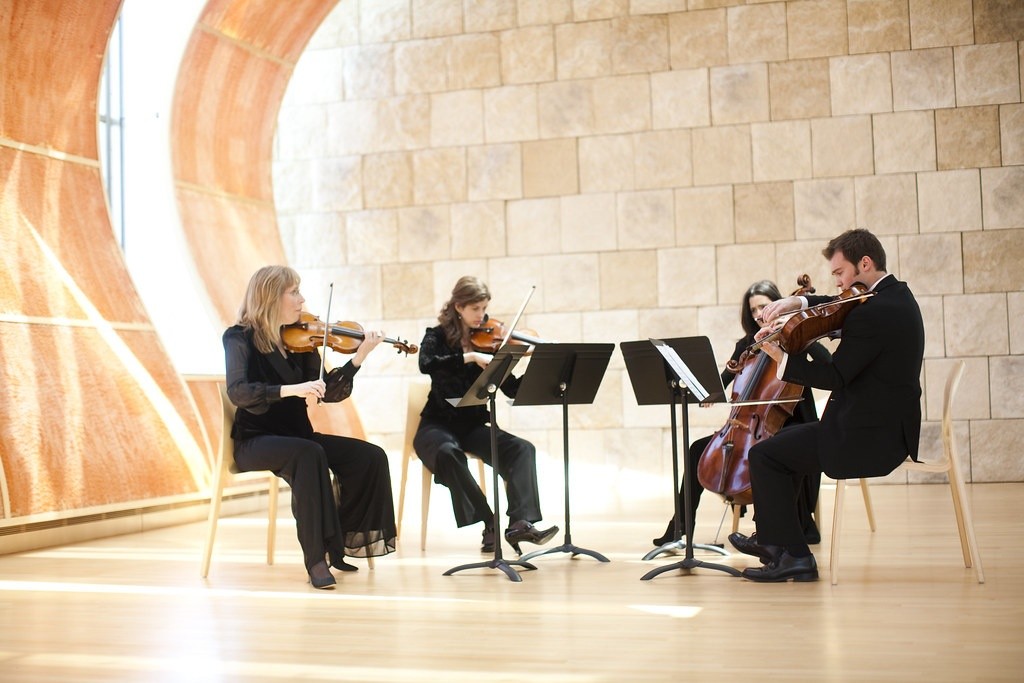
481;517;495;552
304;559;337;589
653;521;695;547
505;524;560;556
325;548;358;571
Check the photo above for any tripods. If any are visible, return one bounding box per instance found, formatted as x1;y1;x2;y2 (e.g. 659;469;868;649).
442;336;744;582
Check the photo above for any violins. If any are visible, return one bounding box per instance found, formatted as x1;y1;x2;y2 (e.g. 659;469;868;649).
469;313;551;357
725;281;869;374
280;311;418;357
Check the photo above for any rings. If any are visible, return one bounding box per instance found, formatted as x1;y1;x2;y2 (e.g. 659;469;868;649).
768;306;770;310
774;311;780;317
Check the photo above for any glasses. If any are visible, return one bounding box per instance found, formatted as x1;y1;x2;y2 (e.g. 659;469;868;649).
281;290;300;297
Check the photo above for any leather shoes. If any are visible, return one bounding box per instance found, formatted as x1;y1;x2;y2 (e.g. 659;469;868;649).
742;550;819;582
728;532;782;566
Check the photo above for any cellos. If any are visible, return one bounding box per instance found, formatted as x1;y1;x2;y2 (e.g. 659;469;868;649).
697;273;816;544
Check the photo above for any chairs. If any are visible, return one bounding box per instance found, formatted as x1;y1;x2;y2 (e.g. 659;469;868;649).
732;361;986;584
397;383;488;550
201;383;382;583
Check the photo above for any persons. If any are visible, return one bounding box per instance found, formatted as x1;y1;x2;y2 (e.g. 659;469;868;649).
728;228;924;582
653;280;832;547
413;276;559;555
223;266;397;590
